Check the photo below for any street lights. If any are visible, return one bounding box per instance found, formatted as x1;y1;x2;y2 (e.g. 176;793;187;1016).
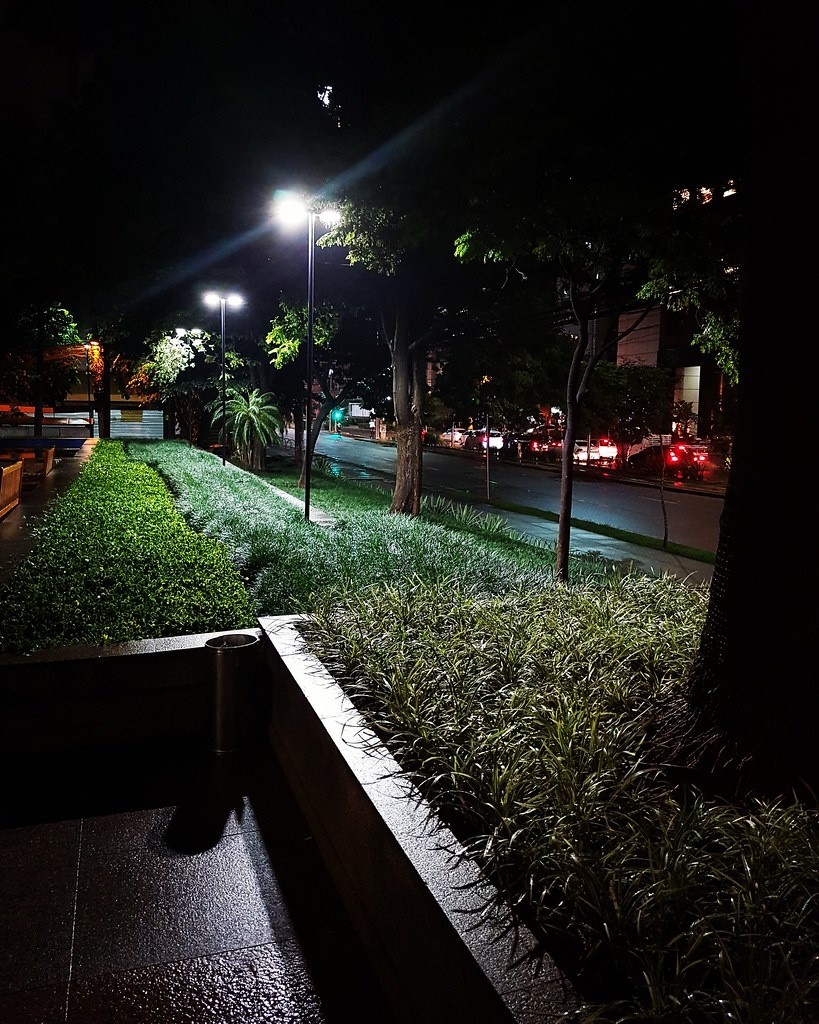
200;287;247;468
83;343;94;438
268;186;346;523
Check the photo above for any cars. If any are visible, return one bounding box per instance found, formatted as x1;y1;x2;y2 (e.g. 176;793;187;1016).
628;444;688;474
441;427;618;462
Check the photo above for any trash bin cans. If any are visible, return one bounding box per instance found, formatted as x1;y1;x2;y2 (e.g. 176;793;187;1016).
203;634;262;754
